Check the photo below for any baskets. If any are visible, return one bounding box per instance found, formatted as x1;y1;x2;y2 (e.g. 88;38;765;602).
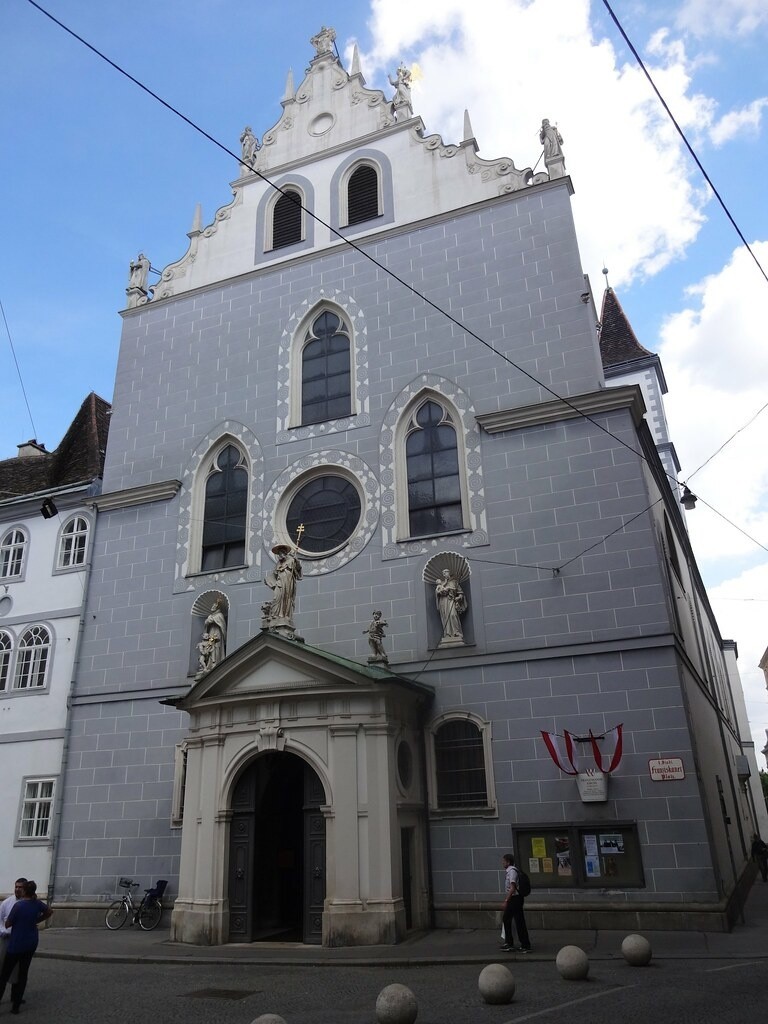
119;878;133;887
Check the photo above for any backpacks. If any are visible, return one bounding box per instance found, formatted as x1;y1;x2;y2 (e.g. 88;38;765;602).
513;866;531;896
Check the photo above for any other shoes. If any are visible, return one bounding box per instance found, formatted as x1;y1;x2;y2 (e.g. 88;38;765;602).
500;943;515;952
517;945;528;950
11;994;25;1004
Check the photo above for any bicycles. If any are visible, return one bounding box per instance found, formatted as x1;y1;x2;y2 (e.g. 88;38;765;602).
105;878;168;931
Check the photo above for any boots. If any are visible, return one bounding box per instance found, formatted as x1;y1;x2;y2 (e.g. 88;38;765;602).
10;992;23;1014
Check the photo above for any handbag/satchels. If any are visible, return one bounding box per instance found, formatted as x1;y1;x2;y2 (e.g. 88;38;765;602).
501;922;506;940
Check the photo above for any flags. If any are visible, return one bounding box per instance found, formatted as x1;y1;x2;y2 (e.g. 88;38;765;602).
540;722;624;775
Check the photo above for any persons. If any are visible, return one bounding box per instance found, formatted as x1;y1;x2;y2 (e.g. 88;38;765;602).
0;878;53;1015
240;126;257;162
388;68;414;113
126;252;149;294
362;610;389;657
500;853;533;954
539;118;563;158
261;543;303;640
196;603;226;674
312;27;336;53
435;569;467;638
